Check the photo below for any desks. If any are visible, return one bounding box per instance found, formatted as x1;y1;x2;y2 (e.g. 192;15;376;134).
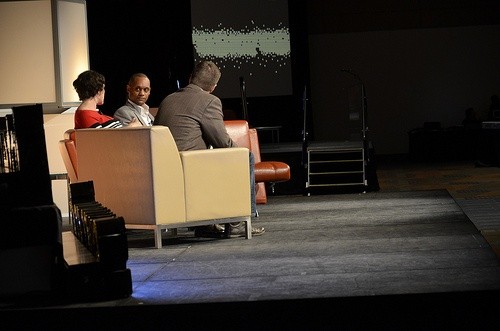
408;128;500;169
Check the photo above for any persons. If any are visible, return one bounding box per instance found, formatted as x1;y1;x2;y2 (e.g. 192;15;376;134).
463;108;478;124
114;73;155;126
74;71;141;129
153;60;264;239
489;95;500;113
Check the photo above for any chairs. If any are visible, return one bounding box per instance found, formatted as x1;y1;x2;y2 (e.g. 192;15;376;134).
59;129;79;224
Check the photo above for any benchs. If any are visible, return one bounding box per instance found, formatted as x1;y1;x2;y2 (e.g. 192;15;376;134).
75;124;252;249
219;121;292;204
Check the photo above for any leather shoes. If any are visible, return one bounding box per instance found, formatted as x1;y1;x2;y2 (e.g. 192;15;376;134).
194;224;225;237
225;221;265;237
